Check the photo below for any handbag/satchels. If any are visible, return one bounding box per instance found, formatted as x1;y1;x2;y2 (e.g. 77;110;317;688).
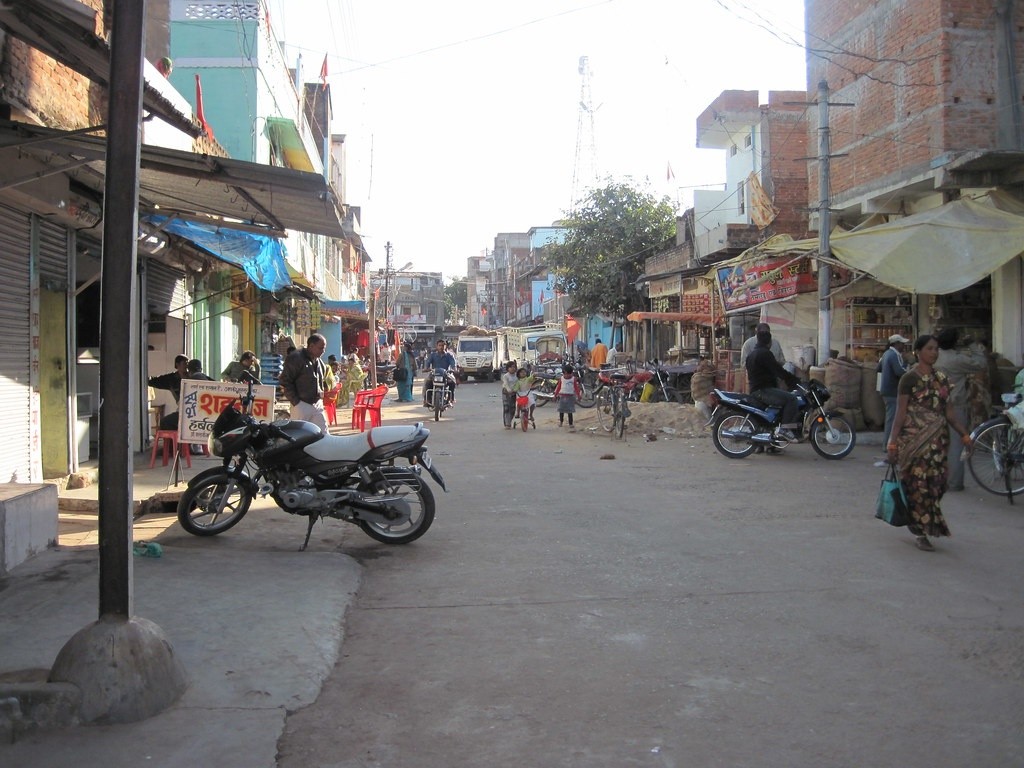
393;368;407;381
875;463;912;526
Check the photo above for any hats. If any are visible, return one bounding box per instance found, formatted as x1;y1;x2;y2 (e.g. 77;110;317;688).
889;334;909;344
938;327;959;350
757;331;771;343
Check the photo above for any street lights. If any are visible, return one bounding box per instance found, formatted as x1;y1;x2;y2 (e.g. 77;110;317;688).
383;262;415;324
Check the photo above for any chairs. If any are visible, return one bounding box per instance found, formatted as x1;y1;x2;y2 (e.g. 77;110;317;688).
323;383;342;427
351;384;388;433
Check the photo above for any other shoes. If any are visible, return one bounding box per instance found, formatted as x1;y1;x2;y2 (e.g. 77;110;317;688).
917;536;935;551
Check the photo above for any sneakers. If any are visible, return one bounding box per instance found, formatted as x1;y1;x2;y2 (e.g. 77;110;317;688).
778;429;798;443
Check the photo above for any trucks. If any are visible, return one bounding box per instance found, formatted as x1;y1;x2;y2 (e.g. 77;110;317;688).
502;325;570;370
456;334;507;384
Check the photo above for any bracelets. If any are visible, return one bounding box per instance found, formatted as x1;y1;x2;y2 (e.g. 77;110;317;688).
961;434;971;443
887;445;897;450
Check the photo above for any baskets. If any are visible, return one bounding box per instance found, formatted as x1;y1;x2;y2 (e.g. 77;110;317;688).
517;397;528;405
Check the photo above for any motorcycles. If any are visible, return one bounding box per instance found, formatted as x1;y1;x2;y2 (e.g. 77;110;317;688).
626;357;686;405
705;378;857;460
365;363;398;388
419;367;459;421
530;362;599;408
175;371;447;554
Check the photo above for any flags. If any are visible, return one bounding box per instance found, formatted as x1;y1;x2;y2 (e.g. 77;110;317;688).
319;55;327;91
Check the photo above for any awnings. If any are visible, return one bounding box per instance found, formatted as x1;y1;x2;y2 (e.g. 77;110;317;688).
0;0;346;325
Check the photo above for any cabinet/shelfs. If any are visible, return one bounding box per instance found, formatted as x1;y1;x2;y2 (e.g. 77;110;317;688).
843;297;917;362
927;276;992;345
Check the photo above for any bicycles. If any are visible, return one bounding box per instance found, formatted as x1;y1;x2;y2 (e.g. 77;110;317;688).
595;367;633;439
510;386;543;432
964;392;1024;507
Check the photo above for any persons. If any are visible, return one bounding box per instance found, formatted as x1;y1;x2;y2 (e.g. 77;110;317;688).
220;351;261;383
278;333;330;435
887;334;972;552
590;338;608;368
605;342;622;364
932;329;987;491
502;361;518;429
394;344;418;402
380;342;391;365
552;365;581;429
415;346;437;369
147;354;216;456
512;368;536;421
876;335;909;453
422;340;458;407
741;322;800;455
322;348;370;408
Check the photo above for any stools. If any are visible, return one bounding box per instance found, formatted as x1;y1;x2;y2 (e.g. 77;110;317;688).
149;426;191;471
